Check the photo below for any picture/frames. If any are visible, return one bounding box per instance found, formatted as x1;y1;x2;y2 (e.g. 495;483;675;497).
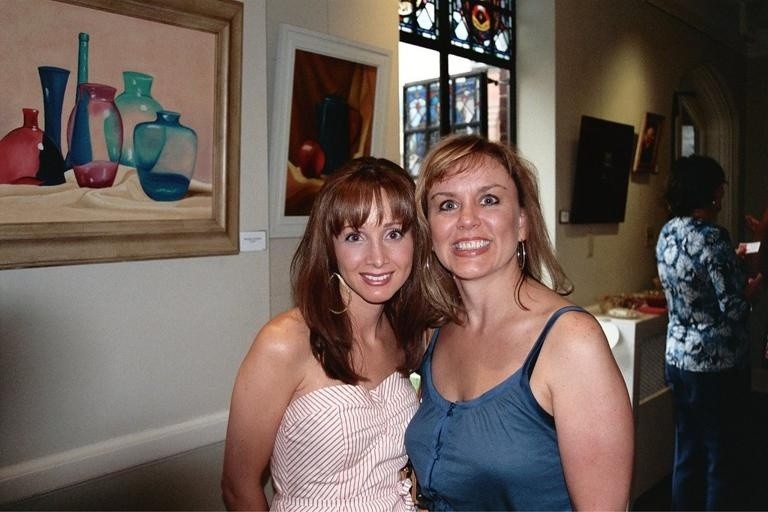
0;0;244;272
569;112;663;223
268;20;393;241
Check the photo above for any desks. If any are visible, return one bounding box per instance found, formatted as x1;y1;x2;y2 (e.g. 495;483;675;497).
580;302;676;512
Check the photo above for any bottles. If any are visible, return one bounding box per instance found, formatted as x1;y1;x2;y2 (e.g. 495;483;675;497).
0;32;199;202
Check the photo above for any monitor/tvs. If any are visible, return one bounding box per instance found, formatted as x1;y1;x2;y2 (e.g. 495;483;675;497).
570;114;634;225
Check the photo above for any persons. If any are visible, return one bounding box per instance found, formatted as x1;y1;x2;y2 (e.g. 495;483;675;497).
220;156;431;512
402;135;637;511
655;155;768;512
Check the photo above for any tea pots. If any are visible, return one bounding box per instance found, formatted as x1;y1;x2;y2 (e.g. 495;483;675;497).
310;93;364;175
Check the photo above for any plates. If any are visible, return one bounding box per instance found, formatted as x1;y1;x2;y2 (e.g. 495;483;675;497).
596;318;621;349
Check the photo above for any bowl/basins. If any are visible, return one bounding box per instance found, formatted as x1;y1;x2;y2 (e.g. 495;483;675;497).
600;290;667;319
